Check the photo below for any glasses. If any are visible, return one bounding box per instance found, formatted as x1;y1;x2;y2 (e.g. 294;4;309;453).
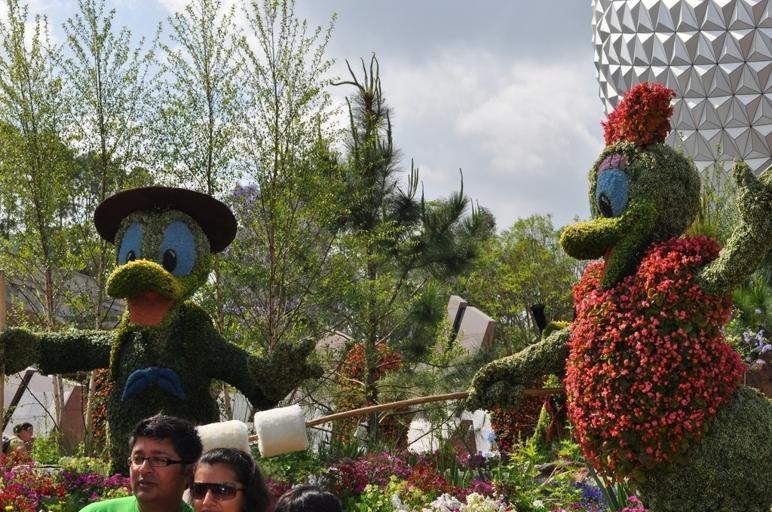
189;481;245;500
127;454;184;468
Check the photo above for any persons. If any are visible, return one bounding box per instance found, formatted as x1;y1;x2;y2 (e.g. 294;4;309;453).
78;414;203;512
191;447;274;512
0;423;33;457
275;486;341;512
